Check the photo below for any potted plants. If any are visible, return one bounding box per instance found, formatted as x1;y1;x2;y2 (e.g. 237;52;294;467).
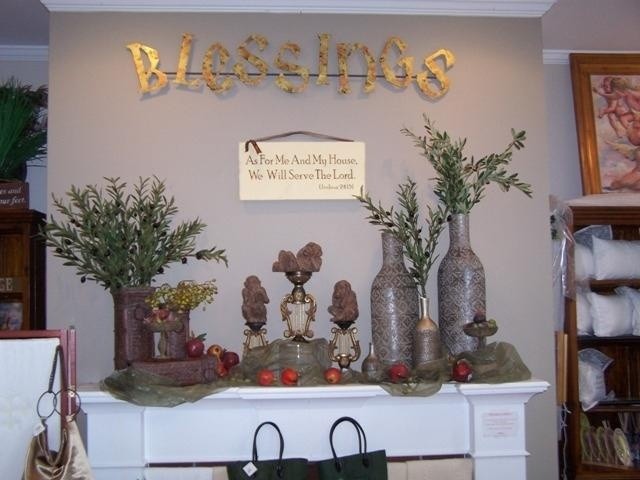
400;113;534;357
32;175;229;371
351;175;456;367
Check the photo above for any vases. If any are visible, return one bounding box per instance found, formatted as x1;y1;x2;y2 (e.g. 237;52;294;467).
371;232;419;381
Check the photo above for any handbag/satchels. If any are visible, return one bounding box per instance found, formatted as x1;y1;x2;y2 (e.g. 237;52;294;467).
319;416;388;480
225;421;308;480
22;343;91;480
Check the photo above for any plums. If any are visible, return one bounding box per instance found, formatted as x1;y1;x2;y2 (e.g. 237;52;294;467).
474;311;485;323
221;352;239;369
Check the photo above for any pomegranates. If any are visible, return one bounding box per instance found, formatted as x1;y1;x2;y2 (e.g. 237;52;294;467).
187;337;204;357
207;344;223;358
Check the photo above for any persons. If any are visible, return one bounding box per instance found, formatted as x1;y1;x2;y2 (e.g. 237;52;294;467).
593;76;640;189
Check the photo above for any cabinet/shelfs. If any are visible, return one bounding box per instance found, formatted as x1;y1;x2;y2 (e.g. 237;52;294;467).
568;205;640;480
0;210;46;332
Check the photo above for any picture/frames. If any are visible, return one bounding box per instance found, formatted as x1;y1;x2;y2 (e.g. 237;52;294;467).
569;53;640;196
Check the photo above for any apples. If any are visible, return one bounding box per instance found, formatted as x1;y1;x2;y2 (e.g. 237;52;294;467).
454;360;472;382
324;368;341;384
281;368;298;385
257;370;273;385
390;363;411;384
